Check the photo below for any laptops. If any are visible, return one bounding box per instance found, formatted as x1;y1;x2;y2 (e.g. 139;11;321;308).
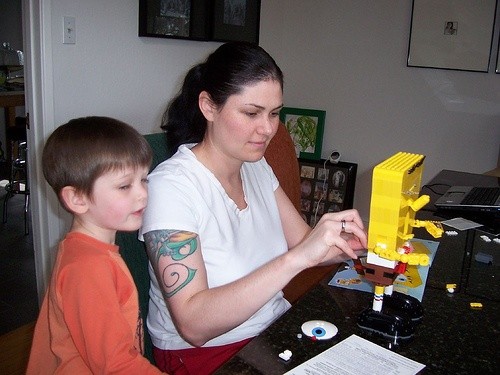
434;186;500;212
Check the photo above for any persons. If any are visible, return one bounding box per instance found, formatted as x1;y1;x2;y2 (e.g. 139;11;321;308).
137;39;368;375
24;117;165;375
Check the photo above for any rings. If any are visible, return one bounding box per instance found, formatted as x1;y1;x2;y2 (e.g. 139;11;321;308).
342;219;345;233
